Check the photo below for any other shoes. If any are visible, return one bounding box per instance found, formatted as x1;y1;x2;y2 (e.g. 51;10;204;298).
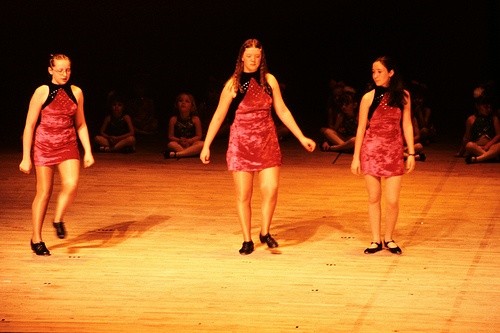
163;151;177;159
464;154;478;164
96;145;106;152
259;232;278;247
239;239;254;254
364;241;382;253
416;153;425;161
53;219;66;238
30;239;49;255
384;239;401;253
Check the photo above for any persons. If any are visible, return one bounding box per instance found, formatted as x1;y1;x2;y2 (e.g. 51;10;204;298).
320;78;500;164
199;39;317;255
18;55;95;256
350;54;415;256
90;80;296;158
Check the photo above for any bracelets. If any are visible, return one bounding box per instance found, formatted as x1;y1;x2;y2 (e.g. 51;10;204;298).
409;154;416;156
